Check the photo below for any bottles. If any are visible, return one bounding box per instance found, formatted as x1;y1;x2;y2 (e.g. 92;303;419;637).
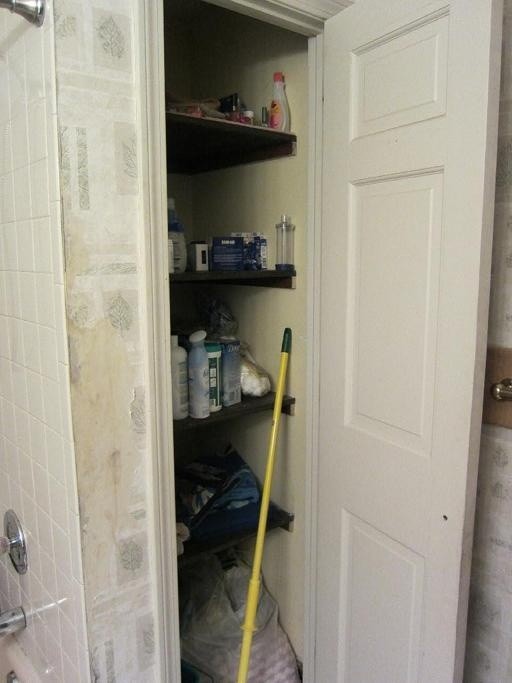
170;336;190;422
273;212;295;270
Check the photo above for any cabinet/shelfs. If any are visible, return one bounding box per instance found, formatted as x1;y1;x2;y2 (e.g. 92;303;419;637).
166;111;296;568
